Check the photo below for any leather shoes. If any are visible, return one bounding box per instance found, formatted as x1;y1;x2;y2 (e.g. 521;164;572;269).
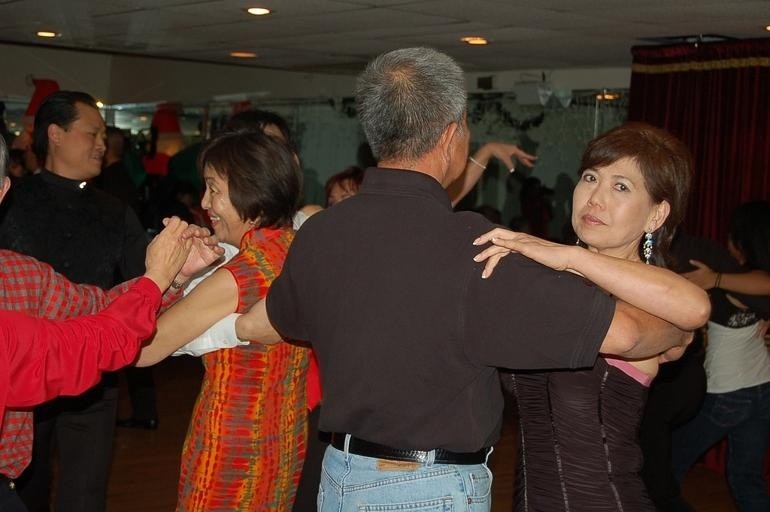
118;414;159;432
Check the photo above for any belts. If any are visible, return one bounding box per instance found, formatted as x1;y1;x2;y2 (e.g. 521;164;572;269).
330;432;487;466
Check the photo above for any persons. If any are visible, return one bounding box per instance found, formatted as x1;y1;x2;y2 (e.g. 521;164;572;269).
1;48;769;512
